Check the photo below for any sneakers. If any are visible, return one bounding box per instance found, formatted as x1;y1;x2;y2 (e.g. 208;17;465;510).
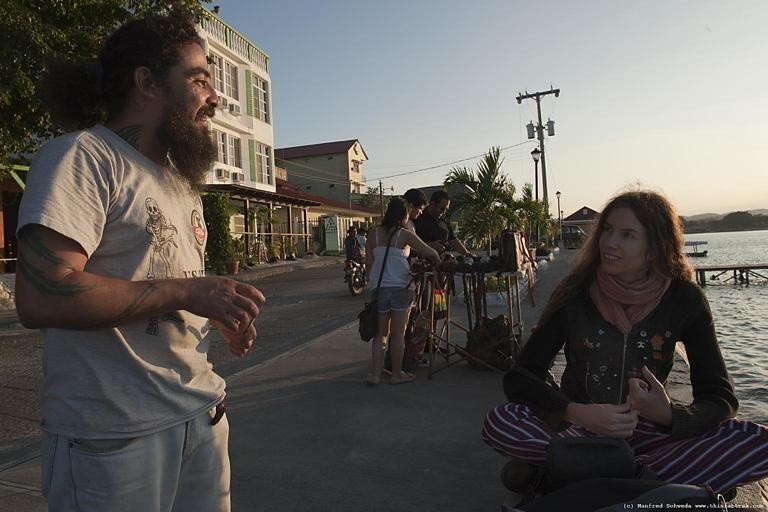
390;373;416;384
367;373;380;385
500;461;559;496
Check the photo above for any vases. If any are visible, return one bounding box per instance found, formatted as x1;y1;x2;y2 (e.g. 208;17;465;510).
225;259;240;275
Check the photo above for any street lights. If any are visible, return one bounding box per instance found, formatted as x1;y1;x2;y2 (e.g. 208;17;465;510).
531;147;542;202
556;191;562;220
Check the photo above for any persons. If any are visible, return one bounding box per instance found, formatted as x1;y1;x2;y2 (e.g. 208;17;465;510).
16;14;265;510
482;192;768;498
344;226;365;257
365;189;477;383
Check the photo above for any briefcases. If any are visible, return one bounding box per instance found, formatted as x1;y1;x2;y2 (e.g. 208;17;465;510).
499;229;526;271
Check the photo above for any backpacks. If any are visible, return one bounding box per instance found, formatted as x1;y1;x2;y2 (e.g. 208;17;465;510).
465;314;511;371
501;437;723;511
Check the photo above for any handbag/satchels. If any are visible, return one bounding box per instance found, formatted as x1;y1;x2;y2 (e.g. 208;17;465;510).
357;300;378;342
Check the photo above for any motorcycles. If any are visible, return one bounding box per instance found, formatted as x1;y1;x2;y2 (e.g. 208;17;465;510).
343;258;367;296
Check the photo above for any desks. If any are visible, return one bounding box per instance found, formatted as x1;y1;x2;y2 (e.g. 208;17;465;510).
411;253;527;377
